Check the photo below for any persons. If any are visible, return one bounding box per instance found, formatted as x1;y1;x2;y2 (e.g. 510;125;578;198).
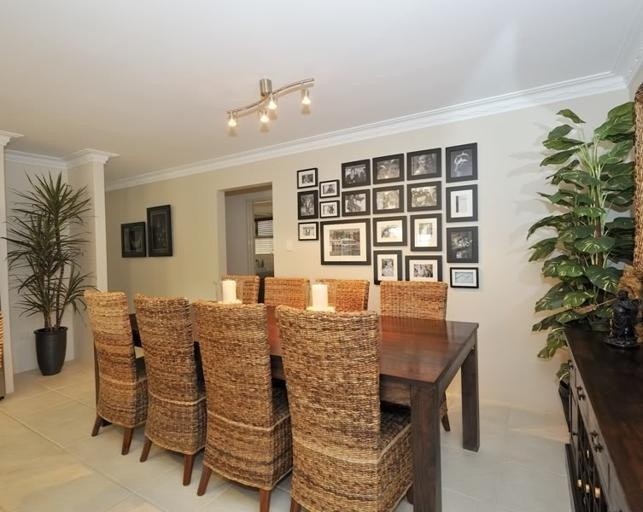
377;162;399;179
346;168;359;184
414;154;435;175
610;291;637;345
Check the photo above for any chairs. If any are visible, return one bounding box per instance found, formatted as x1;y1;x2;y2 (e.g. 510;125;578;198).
131;294;205;485
272;304;413;511
371;280;453;434
218;273;262;303
261;274;311;305
315;278;371;315
188;301;292;510
82;287;150;455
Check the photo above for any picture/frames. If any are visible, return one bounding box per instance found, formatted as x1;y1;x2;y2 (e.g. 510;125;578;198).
296;141;480;290
119;220;145;258
145;203;173;258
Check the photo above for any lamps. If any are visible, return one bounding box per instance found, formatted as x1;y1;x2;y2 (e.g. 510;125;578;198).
222;75;316;131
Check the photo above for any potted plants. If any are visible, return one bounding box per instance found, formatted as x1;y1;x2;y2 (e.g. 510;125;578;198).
524;101;638;438
0;168;103;379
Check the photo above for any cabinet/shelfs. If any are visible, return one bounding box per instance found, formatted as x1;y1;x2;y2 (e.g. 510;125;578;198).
559;327;642;512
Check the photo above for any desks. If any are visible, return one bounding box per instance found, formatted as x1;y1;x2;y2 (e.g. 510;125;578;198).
90;309;481;512
